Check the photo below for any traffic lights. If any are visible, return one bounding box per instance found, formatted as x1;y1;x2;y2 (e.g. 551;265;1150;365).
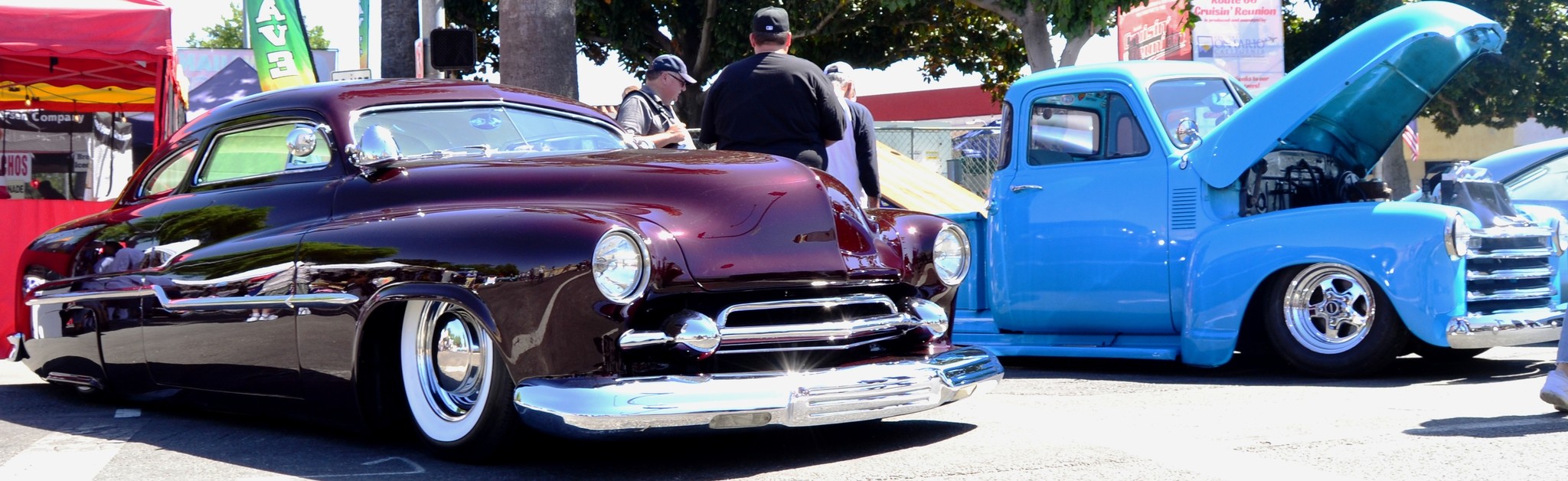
430;28;477;71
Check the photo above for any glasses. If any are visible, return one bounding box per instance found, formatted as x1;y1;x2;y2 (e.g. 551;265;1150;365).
667;72;688;87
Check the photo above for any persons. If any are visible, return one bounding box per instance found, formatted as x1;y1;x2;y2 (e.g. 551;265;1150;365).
817;60;882;210
699;6;846;172
612;54;698;149
36;179;66;200
1538;308;1567;412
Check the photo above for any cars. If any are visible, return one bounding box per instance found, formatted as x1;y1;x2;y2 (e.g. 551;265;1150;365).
893;1;1568;380
0;77;997;465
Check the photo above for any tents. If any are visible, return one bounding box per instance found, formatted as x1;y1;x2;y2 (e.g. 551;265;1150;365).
0;0;188;201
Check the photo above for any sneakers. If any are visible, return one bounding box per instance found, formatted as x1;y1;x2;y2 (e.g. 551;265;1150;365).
1540;369;1568;413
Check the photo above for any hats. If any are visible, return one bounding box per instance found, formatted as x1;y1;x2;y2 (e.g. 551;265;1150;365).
823;61;857;103
648;54;697;83
753;6;790;32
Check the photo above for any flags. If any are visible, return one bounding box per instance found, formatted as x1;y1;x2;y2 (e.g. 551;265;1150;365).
1402;117;1421;164
